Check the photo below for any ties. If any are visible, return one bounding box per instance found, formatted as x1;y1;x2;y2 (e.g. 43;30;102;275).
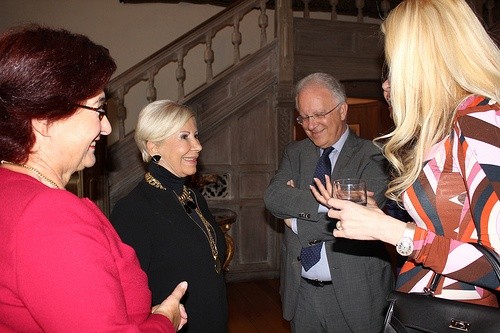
300;146;334;272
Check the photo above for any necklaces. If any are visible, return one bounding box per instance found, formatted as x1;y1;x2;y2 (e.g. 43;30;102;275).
0;158;64;191
142;170;223;274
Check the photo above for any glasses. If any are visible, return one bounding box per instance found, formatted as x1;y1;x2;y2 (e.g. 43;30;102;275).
297;103;341;125
75;102;107;120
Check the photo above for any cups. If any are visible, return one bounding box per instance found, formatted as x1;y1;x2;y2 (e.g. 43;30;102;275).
333;178;367;206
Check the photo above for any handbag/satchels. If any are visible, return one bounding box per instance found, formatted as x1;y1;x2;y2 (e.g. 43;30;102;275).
380;241;500;333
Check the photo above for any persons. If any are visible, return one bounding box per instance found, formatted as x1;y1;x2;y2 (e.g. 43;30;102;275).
108;100;230;333
1;22;188;333
265;71;399;333
310;0;499;333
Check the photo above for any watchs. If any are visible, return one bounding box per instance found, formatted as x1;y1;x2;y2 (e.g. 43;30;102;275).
396;223;419;256
